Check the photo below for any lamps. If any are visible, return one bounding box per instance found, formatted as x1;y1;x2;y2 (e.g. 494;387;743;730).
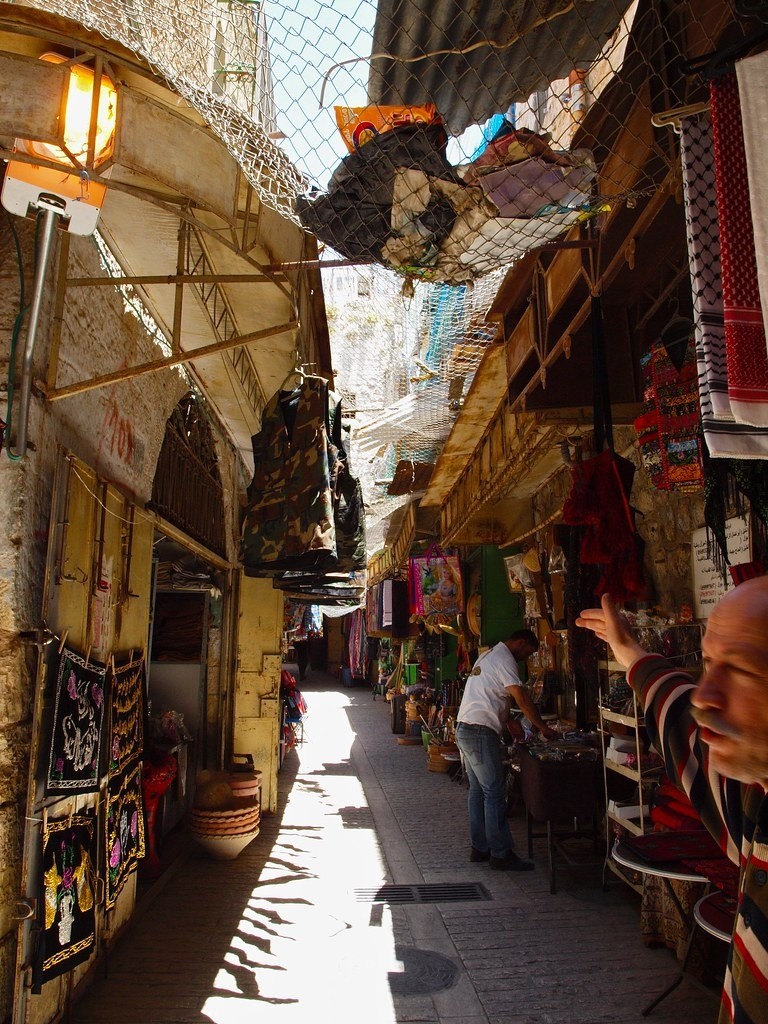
25;51;117;175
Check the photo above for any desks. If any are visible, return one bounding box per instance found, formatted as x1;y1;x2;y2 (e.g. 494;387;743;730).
522;748;603;896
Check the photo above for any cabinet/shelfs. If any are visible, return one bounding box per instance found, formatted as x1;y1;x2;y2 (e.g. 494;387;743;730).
594;653;667;899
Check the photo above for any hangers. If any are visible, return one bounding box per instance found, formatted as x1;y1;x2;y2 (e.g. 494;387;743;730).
676;0;768;78
650;97;714;134
278;349;306;403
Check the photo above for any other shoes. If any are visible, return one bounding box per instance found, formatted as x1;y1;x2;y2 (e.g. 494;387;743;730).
471;848;490;863
487;853;534;870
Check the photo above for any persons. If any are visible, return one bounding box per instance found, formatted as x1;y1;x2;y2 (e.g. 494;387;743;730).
293;642;307;681
576;577;768;1024
456;629;561;869
